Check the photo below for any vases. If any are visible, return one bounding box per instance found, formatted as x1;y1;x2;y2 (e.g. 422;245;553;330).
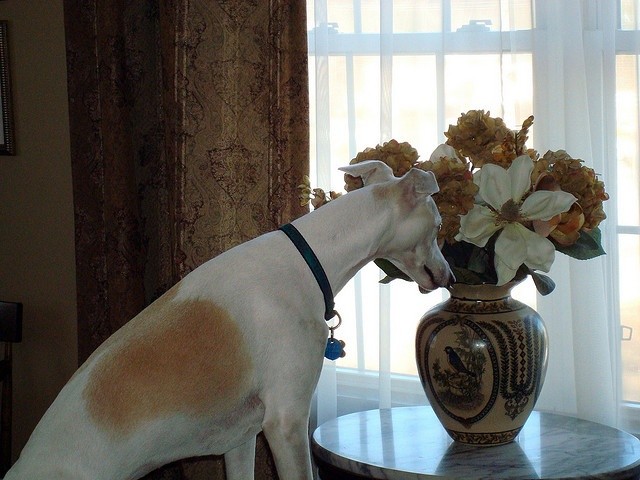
415;276;549;448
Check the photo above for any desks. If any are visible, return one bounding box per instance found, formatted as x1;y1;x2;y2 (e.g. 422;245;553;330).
311;406;640;480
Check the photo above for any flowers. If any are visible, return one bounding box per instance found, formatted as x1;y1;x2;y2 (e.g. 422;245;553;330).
298;109;609;296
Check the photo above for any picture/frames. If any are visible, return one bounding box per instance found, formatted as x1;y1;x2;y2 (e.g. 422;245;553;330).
0;19;16;156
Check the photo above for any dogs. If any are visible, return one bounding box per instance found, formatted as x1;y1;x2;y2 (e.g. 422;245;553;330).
0;159;457;480
